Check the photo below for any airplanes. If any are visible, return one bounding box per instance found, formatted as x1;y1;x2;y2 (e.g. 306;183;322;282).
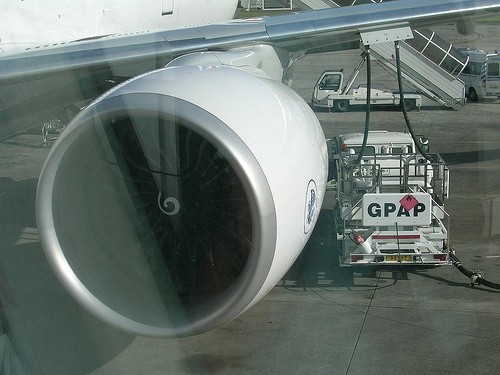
0;0;500;340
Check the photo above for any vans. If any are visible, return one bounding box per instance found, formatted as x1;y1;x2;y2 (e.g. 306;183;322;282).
456;47;500;101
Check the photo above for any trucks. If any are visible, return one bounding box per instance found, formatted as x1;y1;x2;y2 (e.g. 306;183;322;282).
311;70;421;110
326;130;449;269
